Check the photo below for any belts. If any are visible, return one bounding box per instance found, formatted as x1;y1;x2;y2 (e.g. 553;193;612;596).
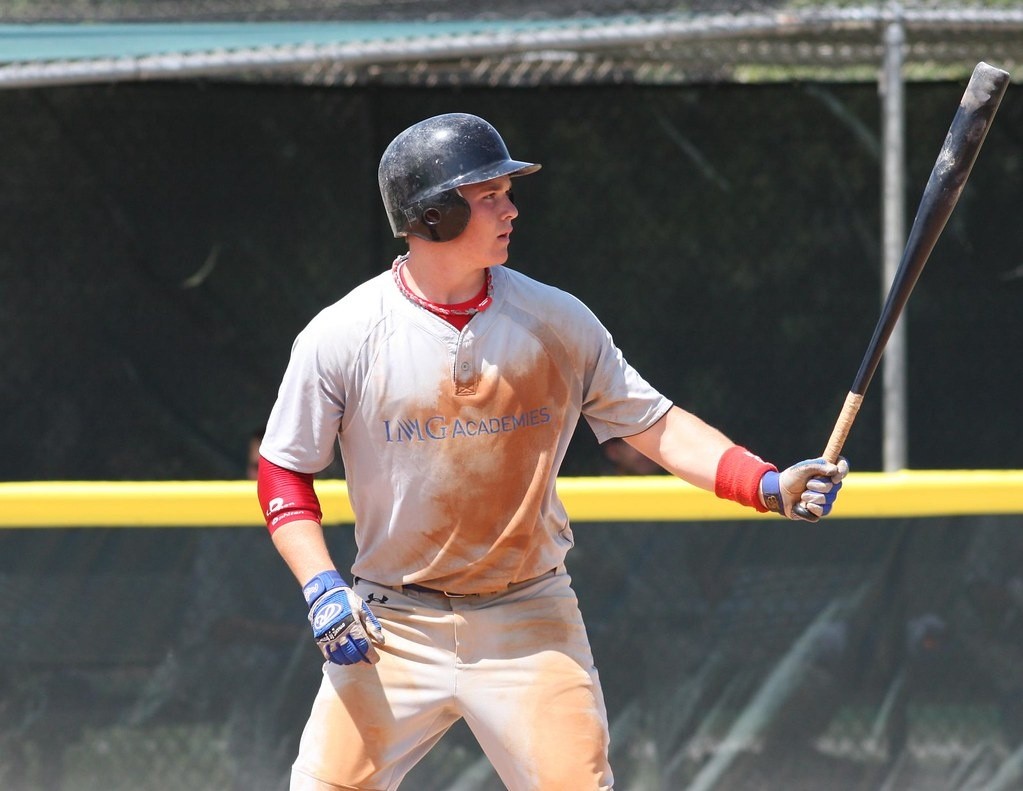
402;583;476;597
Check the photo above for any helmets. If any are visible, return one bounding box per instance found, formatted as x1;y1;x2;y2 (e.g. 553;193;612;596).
377;112;541;242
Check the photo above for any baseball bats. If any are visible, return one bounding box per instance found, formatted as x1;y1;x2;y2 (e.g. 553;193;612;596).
792;60;1014;524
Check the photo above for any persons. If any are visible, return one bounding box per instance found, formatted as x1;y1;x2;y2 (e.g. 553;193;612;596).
603;438;659;474
257;113;849;791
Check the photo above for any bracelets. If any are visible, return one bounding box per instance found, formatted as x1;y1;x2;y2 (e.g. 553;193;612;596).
715;446;778;513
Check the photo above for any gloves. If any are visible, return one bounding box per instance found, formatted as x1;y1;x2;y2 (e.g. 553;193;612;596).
302;570;385;665
761;456;849;524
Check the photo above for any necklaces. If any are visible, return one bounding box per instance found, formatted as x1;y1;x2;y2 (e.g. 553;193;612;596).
393;252;493;314
